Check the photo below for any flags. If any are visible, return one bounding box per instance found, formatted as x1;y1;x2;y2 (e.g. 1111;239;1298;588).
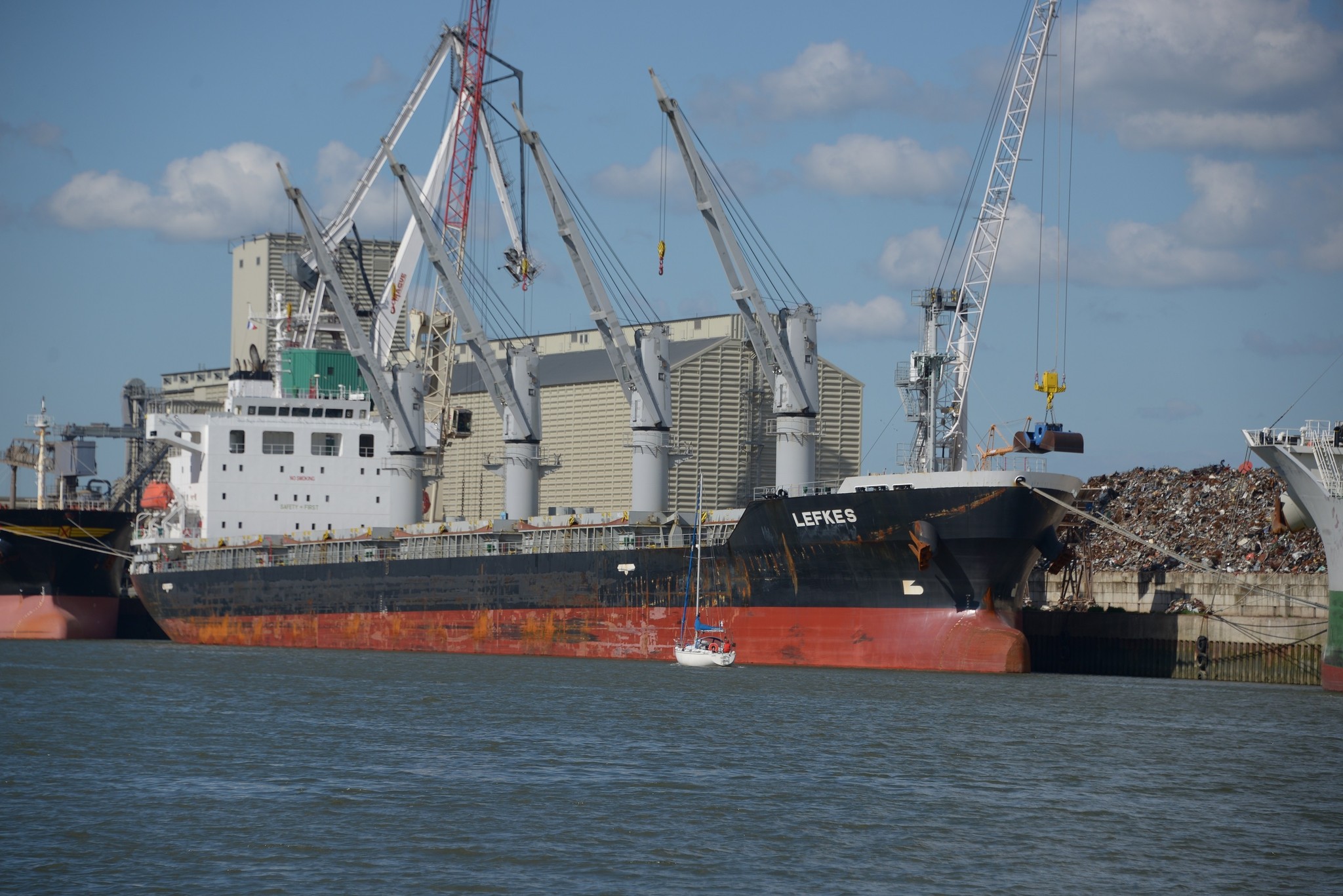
324;532;329;539
489;521;492;528
259;535;263;542
247;322;257;330
368;528;372;536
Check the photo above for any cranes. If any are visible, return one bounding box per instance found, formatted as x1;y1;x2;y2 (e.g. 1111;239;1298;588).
648;66;820;501
275;0;672;528
892;0;1077;473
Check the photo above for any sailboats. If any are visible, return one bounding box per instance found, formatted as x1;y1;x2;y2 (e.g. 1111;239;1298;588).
673;471;736;667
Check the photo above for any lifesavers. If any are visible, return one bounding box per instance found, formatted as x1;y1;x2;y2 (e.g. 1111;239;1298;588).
182;528;192;536
708;643;718;652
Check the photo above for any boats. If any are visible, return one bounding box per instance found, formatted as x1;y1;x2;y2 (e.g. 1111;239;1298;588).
1241;420;1342;694
125;470;1087;673
0;507;131;641
